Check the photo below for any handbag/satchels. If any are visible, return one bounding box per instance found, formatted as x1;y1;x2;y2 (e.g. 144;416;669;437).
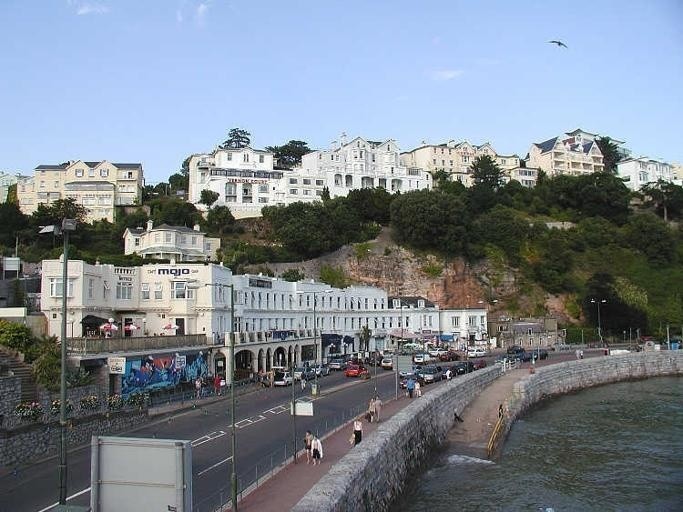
349;434;355;444
418;390;422;396
406;392;409;397
305;446;311;449
365;411;374;422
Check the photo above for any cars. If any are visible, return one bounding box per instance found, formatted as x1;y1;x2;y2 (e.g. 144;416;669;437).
399;348;487;388
271;359;348;387
496;346;548;365
381;358;394;370
345;365;369;377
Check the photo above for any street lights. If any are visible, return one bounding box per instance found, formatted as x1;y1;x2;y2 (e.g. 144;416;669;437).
287;363;297;464
38;218;76;506
296;290;334;384
143;319;147;337
590;299;606;340
109;318;114;337
186;279;237;512
477;300;499;350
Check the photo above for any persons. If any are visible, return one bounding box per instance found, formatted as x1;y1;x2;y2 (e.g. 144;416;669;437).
498;404;504;420
575;347;581;360
300;370;307;392
407;377;421;398
305;430;322;465
257;367;274;389
369;395;384;423
195;374;225;399
446;369;453;381
353;418;363;446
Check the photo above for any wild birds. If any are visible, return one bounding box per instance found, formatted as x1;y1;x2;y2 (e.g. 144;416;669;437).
546;40;568;48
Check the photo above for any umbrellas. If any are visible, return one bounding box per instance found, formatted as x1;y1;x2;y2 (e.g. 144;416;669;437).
96;323;118;337
125;324;141;336
163;322;180;336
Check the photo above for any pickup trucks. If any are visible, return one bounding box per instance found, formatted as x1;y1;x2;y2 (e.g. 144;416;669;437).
637;341;655;349
